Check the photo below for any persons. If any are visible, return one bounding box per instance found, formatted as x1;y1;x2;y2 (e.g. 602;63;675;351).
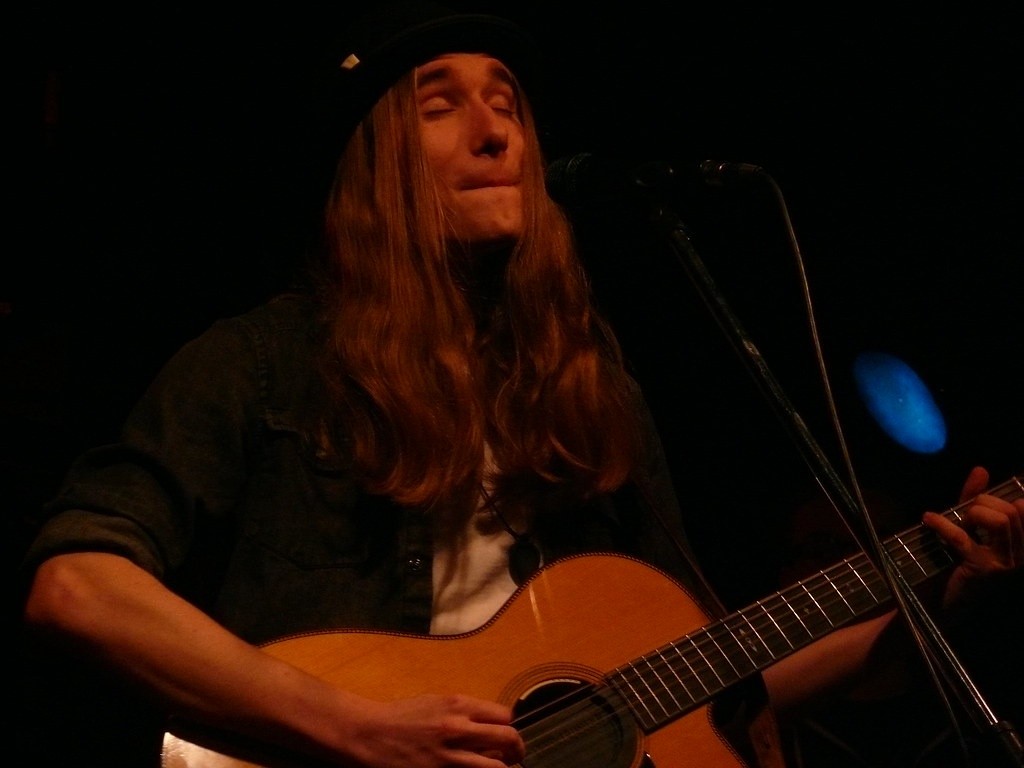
15;16;1024;768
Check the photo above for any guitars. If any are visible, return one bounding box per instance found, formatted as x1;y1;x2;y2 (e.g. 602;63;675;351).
162;474;1024;768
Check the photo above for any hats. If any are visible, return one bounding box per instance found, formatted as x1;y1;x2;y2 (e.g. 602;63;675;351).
319;0;543;138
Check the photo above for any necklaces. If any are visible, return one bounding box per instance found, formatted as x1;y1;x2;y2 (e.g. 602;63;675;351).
479;482;540;587
